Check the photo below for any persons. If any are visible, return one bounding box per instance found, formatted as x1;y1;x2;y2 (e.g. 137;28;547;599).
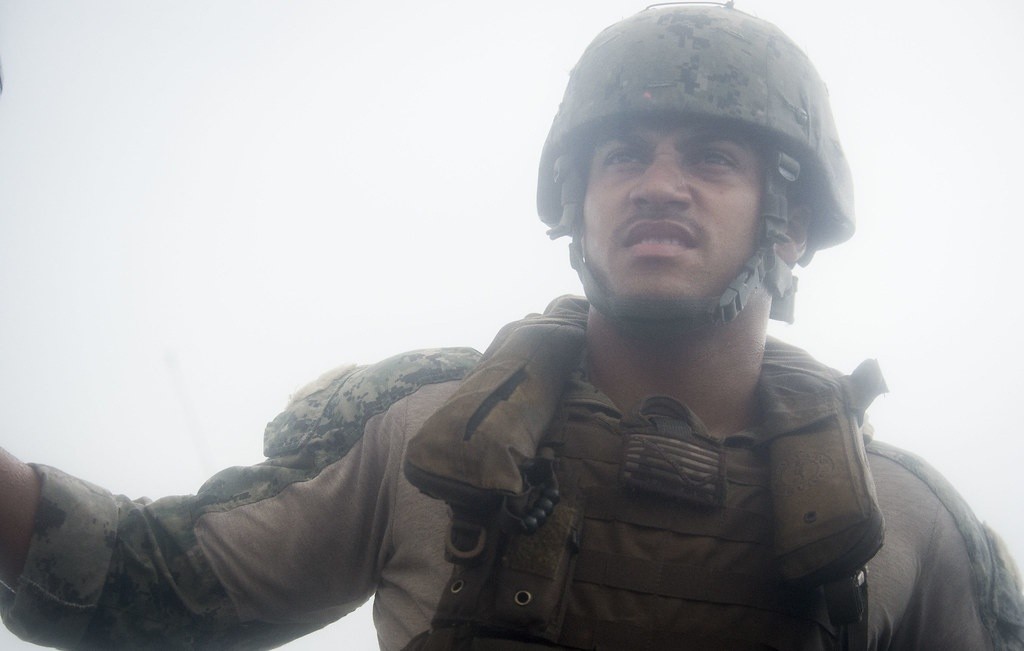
2;1;1024;651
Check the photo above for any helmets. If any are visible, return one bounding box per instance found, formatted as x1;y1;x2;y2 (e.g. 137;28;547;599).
536;4;855;268
